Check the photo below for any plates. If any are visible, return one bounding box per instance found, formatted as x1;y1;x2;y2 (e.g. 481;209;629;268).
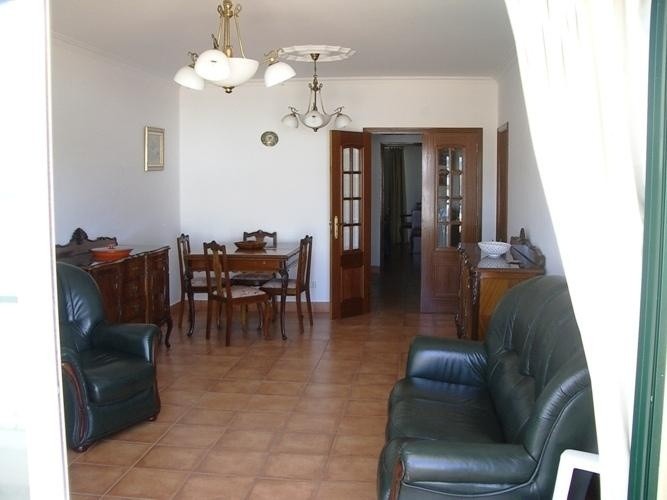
261;131;278;147
233;242;267;249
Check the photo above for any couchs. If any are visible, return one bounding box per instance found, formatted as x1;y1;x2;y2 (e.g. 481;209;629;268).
378;276;602;499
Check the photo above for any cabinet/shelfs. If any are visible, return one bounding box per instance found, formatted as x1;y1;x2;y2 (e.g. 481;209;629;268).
83;245;173;350
454;247;546;341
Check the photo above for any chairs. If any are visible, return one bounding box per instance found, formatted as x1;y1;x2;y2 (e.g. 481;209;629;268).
57;259;162;454
177;230;314;347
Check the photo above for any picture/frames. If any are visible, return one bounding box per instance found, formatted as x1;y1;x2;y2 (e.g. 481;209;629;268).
144;126;164;172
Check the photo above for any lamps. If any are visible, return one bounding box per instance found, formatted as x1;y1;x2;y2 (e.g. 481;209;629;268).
173;0;296;94
278;44;357;133
